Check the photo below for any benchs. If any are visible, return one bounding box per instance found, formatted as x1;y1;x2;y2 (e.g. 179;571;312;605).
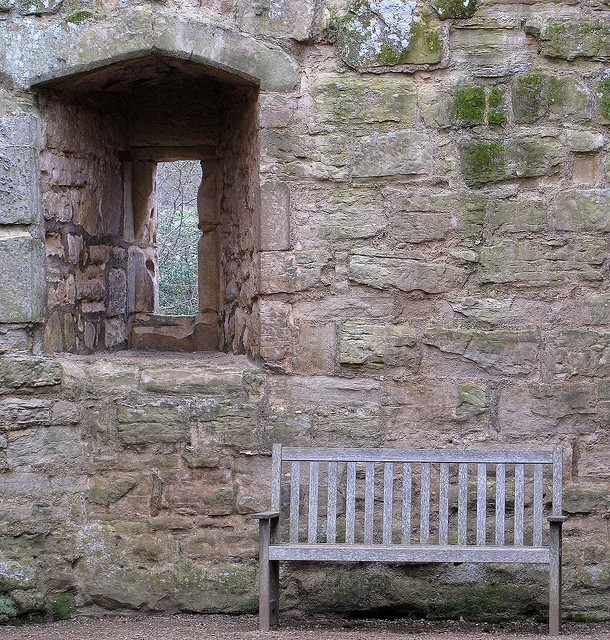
252;442;565;637
